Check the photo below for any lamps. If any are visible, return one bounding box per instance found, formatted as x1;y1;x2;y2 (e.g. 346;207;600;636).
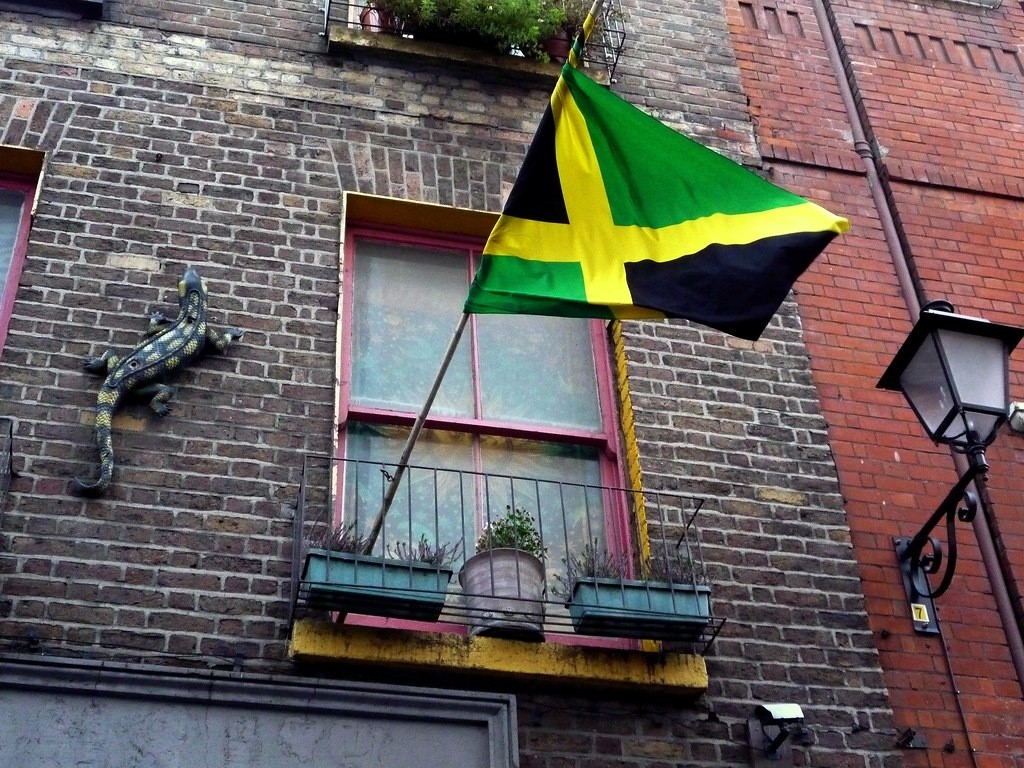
872;299;1024;637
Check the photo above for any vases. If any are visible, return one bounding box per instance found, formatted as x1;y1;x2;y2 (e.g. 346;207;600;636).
359;2;396;35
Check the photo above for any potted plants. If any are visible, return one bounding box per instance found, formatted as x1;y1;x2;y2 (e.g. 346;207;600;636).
549;535;713;639
458;505;549;643
536;0;631;61
300;521;466;619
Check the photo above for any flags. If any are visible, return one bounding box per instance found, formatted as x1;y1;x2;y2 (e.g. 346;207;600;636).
463;14;851;342
